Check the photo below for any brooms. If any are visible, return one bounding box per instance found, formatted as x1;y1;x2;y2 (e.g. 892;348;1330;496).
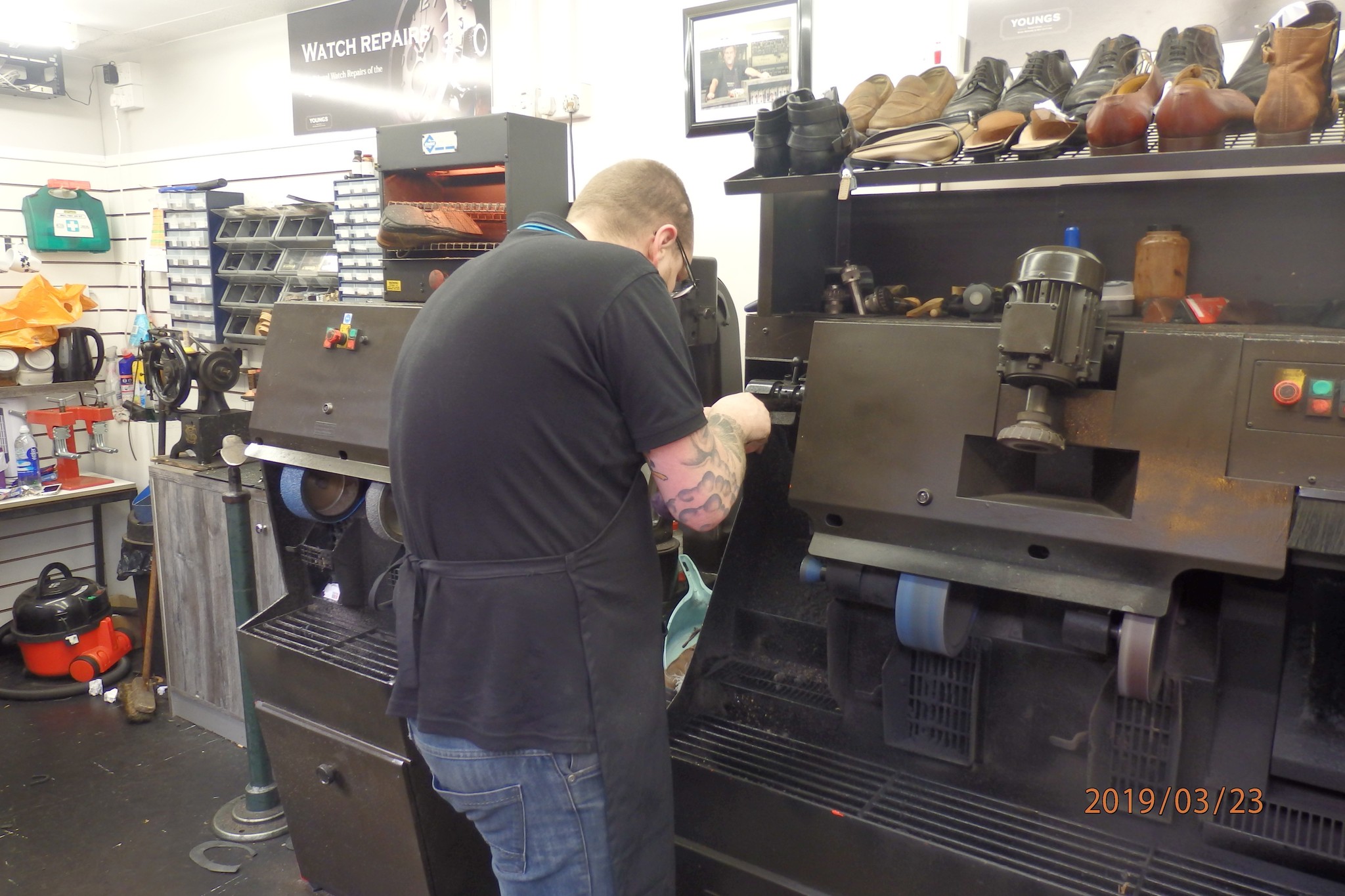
119;541;158;723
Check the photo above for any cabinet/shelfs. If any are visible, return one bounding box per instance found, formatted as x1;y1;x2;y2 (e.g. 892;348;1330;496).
254;698;500;896
0;380;138;586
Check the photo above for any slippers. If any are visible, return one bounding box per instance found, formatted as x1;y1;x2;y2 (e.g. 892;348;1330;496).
963;108;1027;157
1011;109;1086;160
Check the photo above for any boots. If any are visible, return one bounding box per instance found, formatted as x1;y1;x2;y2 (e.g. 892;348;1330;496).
1250;18;1341;146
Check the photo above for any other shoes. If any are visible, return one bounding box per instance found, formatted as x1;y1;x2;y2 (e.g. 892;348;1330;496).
1230;25;1271;104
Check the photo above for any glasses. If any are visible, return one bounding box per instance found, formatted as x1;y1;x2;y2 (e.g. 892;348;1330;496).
654;230;698;299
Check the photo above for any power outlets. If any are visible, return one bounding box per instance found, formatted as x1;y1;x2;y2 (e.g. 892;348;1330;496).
110;85;144;111
112;62;143;87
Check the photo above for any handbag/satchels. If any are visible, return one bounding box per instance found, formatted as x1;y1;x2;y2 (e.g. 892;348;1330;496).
849;119;972;166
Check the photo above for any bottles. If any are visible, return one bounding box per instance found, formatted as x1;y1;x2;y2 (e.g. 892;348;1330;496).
14;425;42;493
362;155;374;177
1134;224;1190;319
352;150;362;178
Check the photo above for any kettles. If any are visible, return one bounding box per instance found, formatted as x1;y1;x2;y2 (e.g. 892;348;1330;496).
52;327;104;383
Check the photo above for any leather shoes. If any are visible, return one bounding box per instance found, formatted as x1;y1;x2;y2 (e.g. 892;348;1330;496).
1155;62;1257;151
786;86;850;175
866;66;958;136
1079;46;1166;156
751;83;813;178
941;58;1014;119
842;73;894;132
1060;34;1140;117
995;49;1078;120
1153;23;1227;95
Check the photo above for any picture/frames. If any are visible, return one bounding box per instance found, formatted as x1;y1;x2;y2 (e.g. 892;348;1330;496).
681;0;813;138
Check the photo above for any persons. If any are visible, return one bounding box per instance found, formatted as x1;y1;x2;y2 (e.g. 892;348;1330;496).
390;160;772;896
707;46;771;100
